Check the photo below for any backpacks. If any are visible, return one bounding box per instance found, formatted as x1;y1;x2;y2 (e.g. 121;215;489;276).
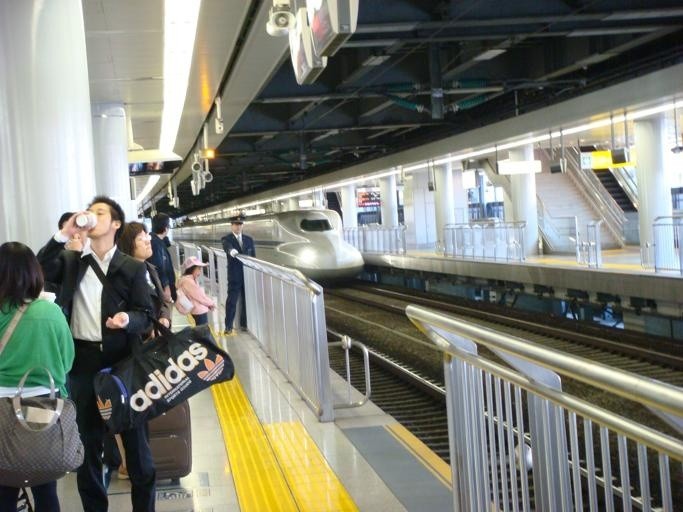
174;284;194;315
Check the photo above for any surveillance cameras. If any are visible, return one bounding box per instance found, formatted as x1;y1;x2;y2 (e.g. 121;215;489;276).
215;119;223;135
671;146;683;154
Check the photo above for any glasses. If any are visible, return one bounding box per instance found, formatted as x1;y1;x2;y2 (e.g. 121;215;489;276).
137;234;152;242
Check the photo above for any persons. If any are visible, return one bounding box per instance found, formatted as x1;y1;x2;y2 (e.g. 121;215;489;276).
41;212;215;477
0;241;76;511
38;196;157;511
220;216;256;336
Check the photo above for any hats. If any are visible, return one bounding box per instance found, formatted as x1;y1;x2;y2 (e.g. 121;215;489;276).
229;215;245;223
183;256;209;270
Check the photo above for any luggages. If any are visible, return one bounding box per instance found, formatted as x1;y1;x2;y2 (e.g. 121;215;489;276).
148;401;192;485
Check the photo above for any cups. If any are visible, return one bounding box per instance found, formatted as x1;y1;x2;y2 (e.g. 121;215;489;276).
76;211;97;229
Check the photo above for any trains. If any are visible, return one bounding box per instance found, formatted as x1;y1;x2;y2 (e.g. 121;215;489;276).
170;210;364;281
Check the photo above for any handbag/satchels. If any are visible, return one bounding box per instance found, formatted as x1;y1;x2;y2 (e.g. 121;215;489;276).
0;366;85;488
93;324;234;434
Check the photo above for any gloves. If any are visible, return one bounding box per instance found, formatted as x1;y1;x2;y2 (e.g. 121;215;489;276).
230;249;239;258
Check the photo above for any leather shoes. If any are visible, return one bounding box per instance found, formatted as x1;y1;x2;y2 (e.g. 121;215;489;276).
239;325;247;330
224;326;232;334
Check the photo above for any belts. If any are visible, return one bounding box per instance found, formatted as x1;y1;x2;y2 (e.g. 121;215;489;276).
79;342;102;350
